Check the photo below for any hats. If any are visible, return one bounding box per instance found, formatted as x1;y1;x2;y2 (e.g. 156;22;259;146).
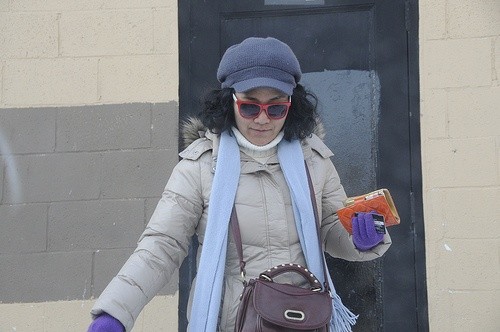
216;35;302;96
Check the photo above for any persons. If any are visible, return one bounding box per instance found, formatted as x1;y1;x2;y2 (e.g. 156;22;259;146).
87;37;393;331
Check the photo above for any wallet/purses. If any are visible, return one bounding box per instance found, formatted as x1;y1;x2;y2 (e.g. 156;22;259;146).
336;188;400;236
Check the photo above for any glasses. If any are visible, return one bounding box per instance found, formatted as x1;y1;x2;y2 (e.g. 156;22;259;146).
232;93;291;120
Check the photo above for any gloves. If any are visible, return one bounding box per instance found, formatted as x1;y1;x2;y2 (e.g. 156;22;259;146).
352;210;385;252
87;312;126;332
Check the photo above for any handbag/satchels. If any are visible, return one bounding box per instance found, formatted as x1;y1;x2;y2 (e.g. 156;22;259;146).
233;263;333;332
